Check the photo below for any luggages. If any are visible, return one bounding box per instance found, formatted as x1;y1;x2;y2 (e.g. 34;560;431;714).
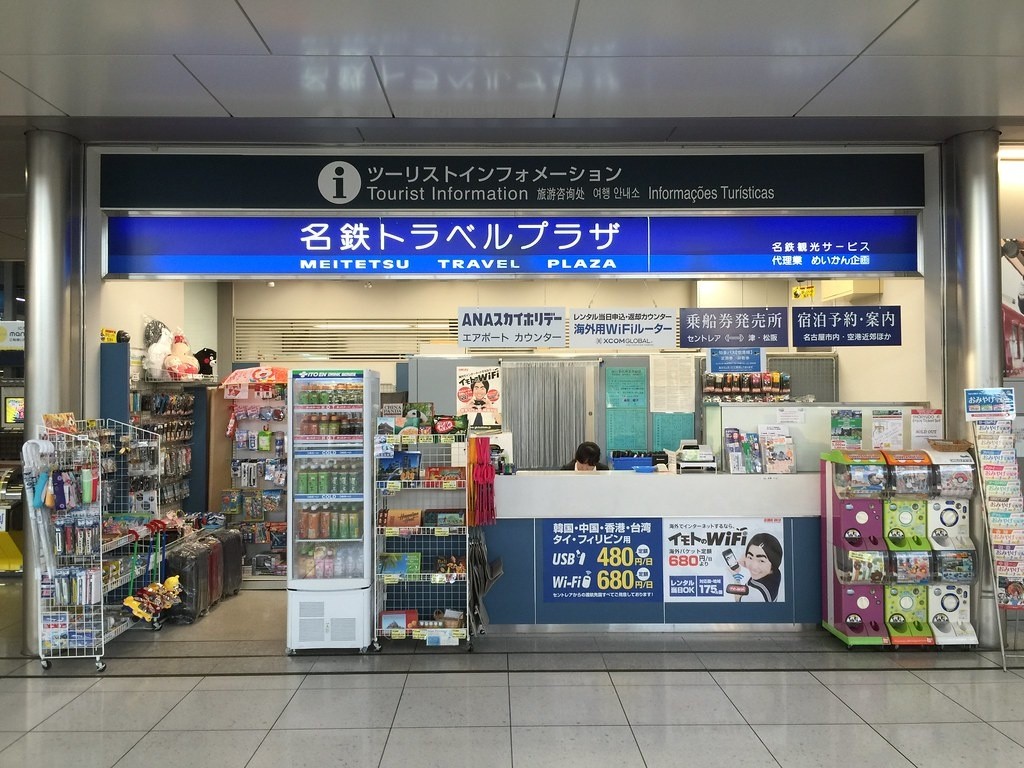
213;529;243;602
196;537;222;607
165;543;210;624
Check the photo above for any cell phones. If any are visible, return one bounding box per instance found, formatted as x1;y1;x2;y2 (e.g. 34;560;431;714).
722;549;739;570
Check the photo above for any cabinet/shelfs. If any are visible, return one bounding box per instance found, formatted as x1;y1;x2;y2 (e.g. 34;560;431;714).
972;419;1024;673
100;342;227;620
373;433;473;654
229;384;287;589
34;417;161;674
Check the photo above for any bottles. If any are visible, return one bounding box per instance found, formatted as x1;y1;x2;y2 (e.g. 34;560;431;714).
295;383;366;578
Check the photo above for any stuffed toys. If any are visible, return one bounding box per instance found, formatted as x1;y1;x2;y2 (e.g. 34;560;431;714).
141;319;174;379
164;333;201;382
193;347;217;378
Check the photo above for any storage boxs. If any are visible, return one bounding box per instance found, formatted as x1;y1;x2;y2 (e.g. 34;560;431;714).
609;456;652;471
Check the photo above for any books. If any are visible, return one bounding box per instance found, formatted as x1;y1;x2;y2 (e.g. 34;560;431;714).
971;419;1024;609
724;424;795;474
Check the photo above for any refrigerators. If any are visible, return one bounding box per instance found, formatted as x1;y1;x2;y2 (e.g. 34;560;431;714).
286;368;382;655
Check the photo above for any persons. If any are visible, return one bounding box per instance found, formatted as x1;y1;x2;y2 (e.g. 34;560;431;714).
561;441;608;471
457;376;502;425
722;531;783;604
